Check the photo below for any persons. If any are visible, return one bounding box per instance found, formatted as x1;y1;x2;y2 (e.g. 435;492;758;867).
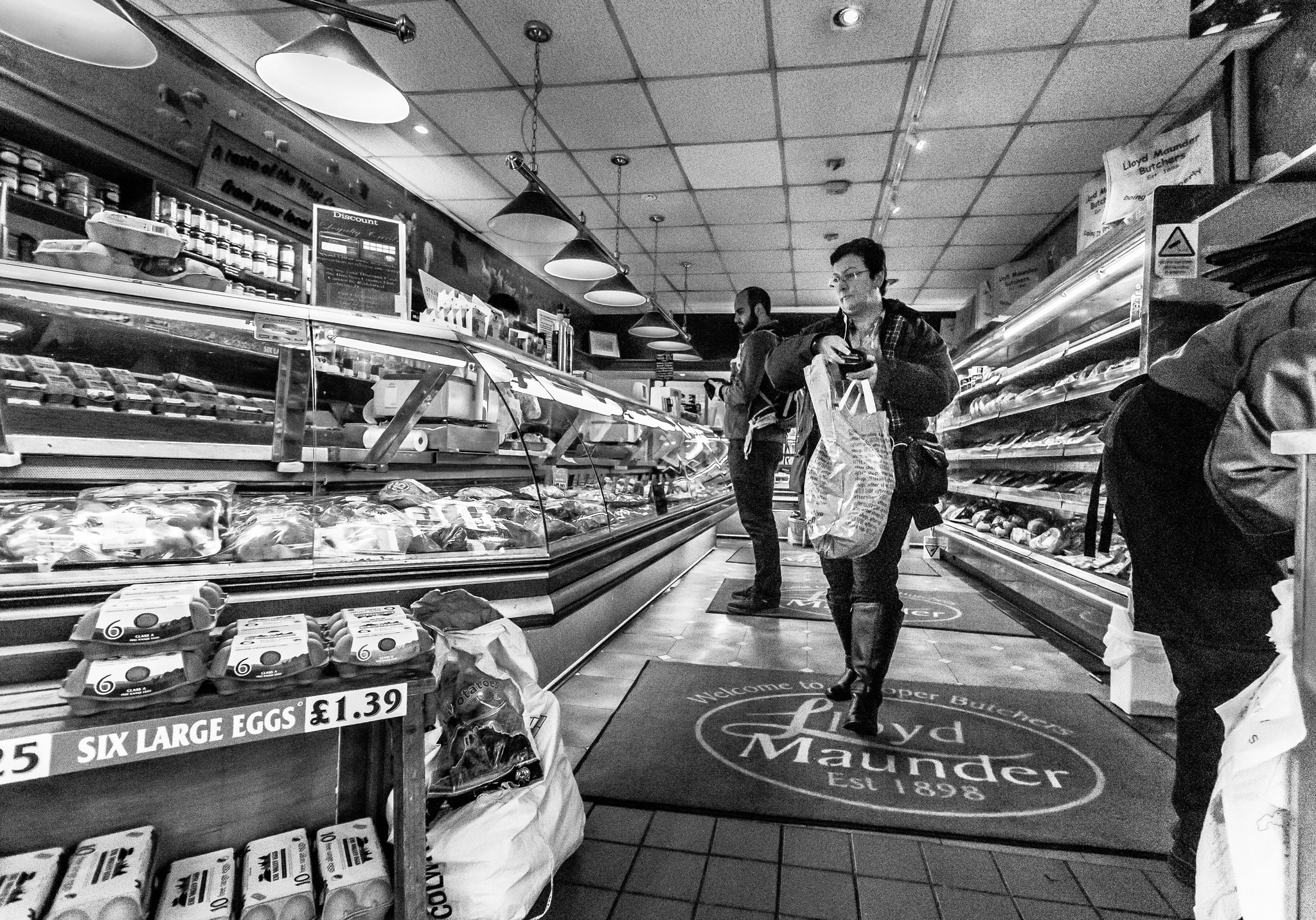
765;237;960;735
1099;278;1316;884
709;287;790;612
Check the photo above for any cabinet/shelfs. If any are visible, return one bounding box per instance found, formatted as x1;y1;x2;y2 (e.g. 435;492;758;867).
1;259;736;920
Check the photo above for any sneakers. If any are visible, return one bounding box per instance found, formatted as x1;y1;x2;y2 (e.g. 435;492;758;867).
727;593;779;613
732;587;755;600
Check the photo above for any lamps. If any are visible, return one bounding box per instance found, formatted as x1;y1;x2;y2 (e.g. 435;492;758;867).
0;0;158;71
888;200;901;214
906;134;926;152
254;11;410;125
488;21;704;362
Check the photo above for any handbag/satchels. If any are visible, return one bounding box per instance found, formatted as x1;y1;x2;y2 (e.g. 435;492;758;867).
801;378;895;561
907;436;950;498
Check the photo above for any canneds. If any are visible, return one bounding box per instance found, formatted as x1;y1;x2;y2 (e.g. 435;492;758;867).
154;192;294;303
0;140;135;221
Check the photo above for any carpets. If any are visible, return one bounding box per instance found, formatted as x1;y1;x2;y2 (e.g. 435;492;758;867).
706;577;1041;639
725;545;941;577
571;659;1179;860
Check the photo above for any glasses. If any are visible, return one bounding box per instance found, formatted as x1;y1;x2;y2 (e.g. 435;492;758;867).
828;270;871;288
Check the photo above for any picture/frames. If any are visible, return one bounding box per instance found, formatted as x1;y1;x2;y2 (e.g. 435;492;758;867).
588;328;621;360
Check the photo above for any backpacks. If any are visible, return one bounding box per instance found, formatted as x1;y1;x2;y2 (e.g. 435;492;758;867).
738;328;803;421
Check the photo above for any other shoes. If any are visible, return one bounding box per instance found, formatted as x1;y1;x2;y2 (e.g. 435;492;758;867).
1168;840;1198;889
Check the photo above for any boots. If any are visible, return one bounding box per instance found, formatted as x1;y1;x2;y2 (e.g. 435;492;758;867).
824;593;859;701
842;600;905;735
